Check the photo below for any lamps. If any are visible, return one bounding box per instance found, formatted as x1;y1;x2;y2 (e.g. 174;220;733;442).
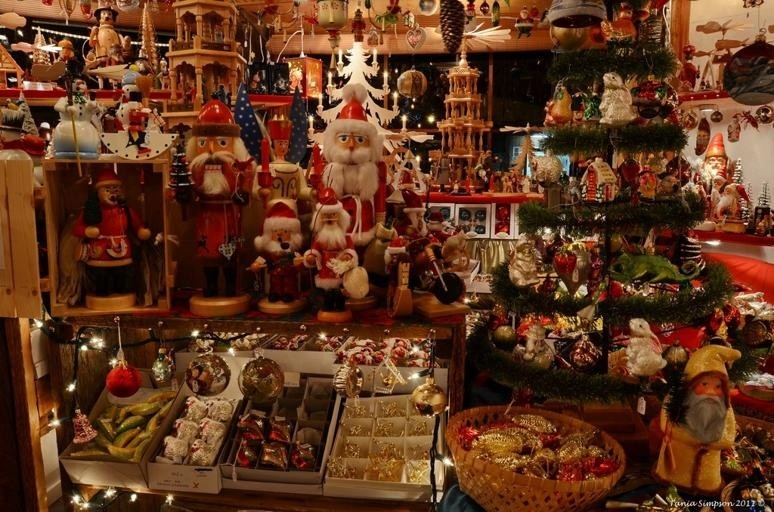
276;30;324;100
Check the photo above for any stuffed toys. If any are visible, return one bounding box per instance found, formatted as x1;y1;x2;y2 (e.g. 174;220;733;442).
0;2;774;511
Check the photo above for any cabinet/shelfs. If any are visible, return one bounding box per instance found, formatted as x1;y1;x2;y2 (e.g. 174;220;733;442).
670;89;774;246
49;306;467;511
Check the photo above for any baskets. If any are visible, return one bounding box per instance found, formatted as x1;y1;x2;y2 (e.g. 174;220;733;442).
441;405;626;512
